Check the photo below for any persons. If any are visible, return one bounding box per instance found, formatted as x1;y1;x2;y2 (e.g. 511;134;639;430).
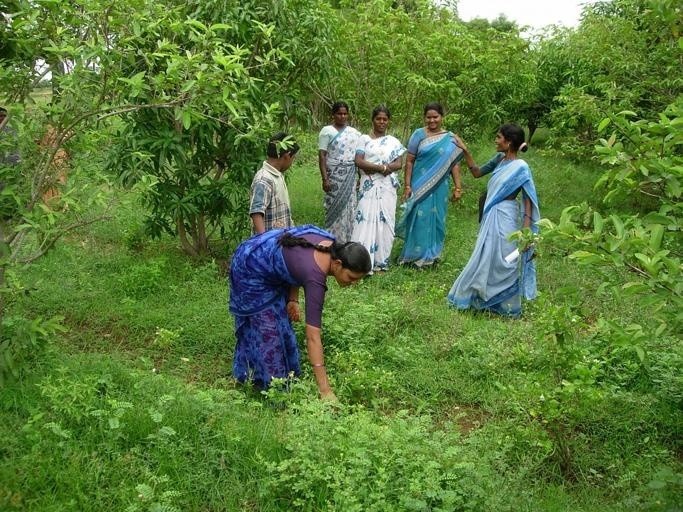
447;124;541;321
318;101;362;246
228;223;372;415
249;132;299;237
395;103;464;268
350;106;408;276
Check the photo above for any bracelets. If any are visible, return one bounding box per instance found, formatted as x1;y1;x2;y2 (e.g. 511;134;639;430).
288;298;300;304
455;187;462;192
322;178;328;181
405;186;411;189
310;363;326;368
382;164;387;174
469;160;475;169
